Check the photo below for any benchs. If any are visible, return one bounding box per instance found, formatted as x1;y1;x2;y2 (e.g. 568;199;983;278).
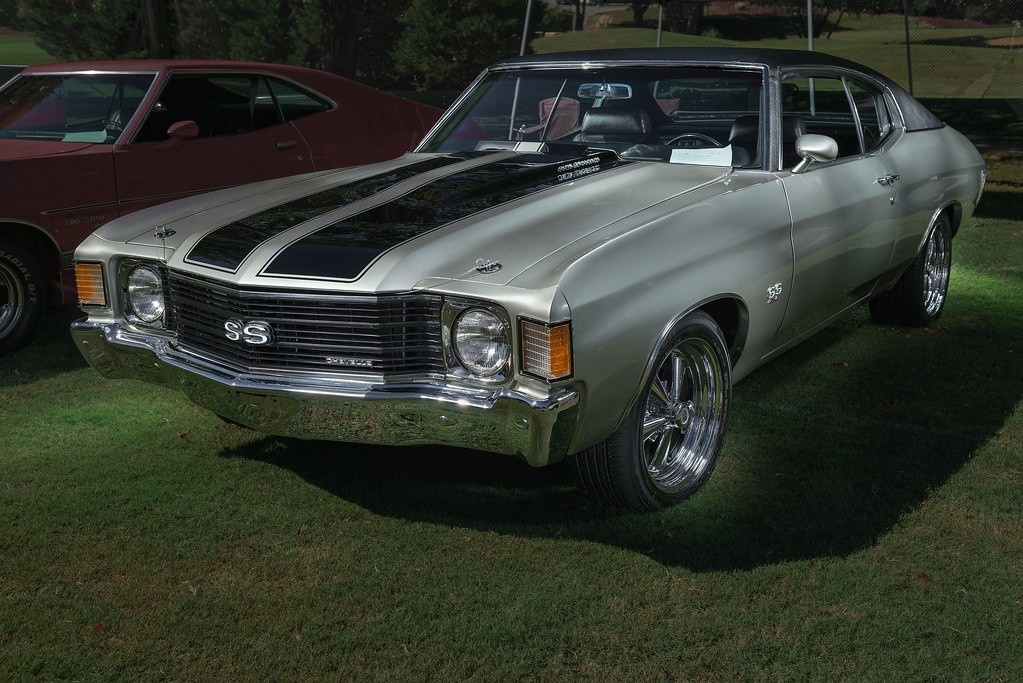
668;122;875;162
155;102;247;138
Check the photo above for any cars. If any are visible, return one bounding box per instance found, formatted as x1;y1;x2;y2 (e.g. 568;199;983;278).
68;45;989;520
0;58;484;359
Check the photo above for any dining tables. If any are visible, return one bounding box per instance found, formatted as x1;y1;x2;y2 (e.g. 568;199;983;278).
566;104;661;163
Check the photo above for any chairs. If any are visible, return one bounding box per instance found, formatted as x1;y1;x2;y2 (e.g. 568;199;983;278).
714;113;810;176
